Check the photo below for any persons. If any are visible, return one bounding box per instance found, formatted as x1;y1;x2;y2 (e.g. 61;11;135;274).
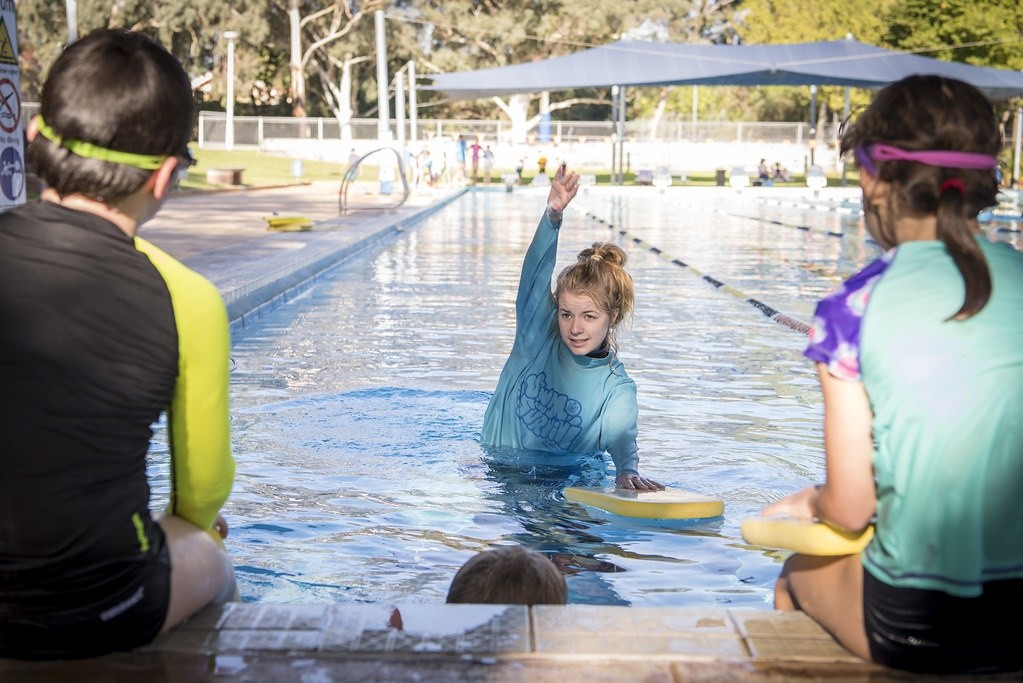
759;159;787;182
404;145;451;185
762;72;1022;676
446;546;567;604
346;149;361;182
482;162;666;491
517;151;566;178
0;27;236;661
456;135;493;184
